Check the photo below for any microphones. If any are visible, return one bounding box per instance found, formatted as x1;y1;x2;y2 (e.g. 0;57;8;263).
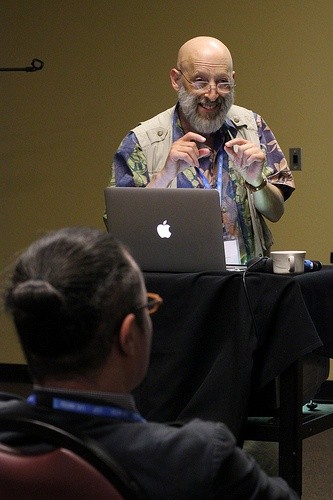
304;259;322;271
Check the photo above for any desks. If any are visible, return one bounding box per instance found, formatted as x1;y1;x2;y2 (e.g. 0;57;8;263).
143;266;333;500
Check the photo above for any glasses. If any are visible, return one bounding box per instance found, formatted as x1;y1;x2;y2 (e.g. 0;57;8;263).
133;292;163;315
178;69;237;94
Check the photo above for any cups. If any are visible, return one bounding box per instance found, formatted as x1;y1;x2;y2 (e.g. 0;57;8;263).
270;250;306;274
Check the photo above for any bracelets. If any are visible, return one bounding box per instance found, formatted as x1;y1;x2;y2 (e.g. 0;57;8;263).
245;175;267;192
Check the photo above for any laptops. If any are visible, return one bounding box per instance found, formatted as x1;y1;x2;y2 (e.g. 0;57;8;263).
106;187;247;273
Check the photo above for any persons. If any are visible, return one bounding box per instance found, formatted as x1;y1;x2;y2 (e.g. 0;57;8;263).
108;35;295;264
0;228;295;500
